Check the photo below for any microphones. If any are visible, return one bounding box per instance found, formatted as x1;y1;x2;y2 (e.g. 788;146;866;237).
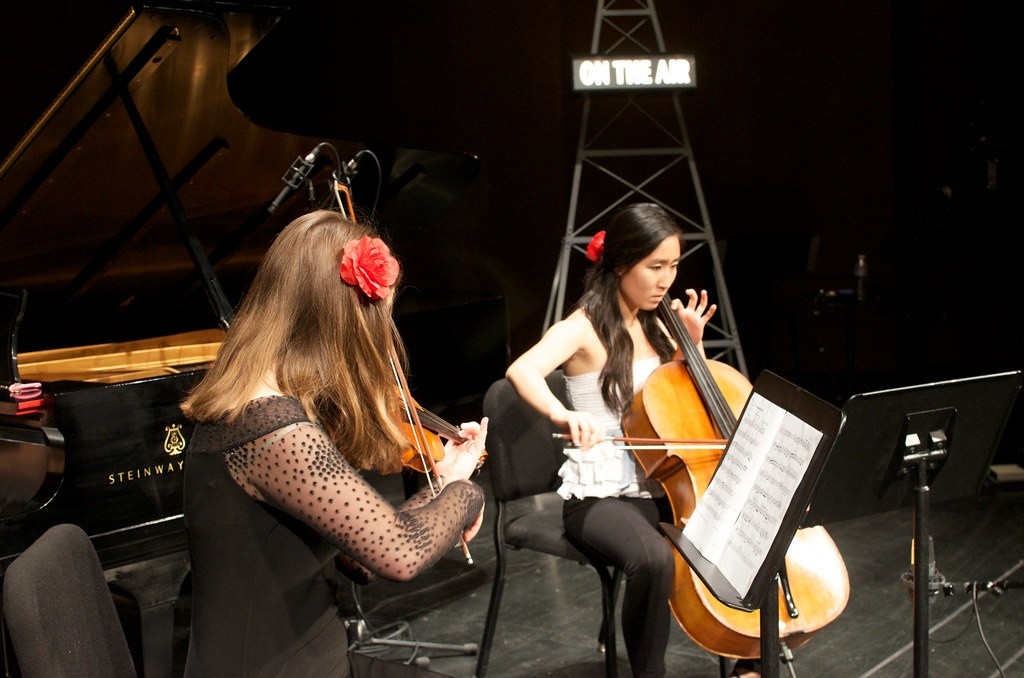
316;151;365;210
268;147;320;214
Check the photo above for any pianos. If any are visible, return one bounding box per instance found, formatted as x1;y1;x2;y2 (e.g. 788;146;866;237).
1;2;360;678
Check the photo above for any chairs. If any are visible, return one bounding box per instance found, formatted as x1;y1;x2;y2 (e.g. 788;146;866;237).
0;524;140;678
474;368;732;678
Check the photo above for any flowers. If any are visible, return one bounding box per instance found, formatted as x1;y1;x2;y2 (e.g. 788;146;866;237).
339;231;399;301
585;230;605;263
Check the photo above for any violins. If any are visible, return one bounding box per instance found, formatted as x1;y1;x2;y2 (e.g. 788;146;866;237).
391;382;491;478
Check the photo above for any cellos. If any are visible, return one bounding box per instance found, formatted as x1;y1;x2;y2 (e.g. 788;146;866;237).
620;283;853;677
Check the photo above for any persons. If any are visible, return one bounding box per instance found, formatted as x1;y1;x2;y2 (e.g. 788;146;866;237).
179;211;489;678
506;203;761;678
